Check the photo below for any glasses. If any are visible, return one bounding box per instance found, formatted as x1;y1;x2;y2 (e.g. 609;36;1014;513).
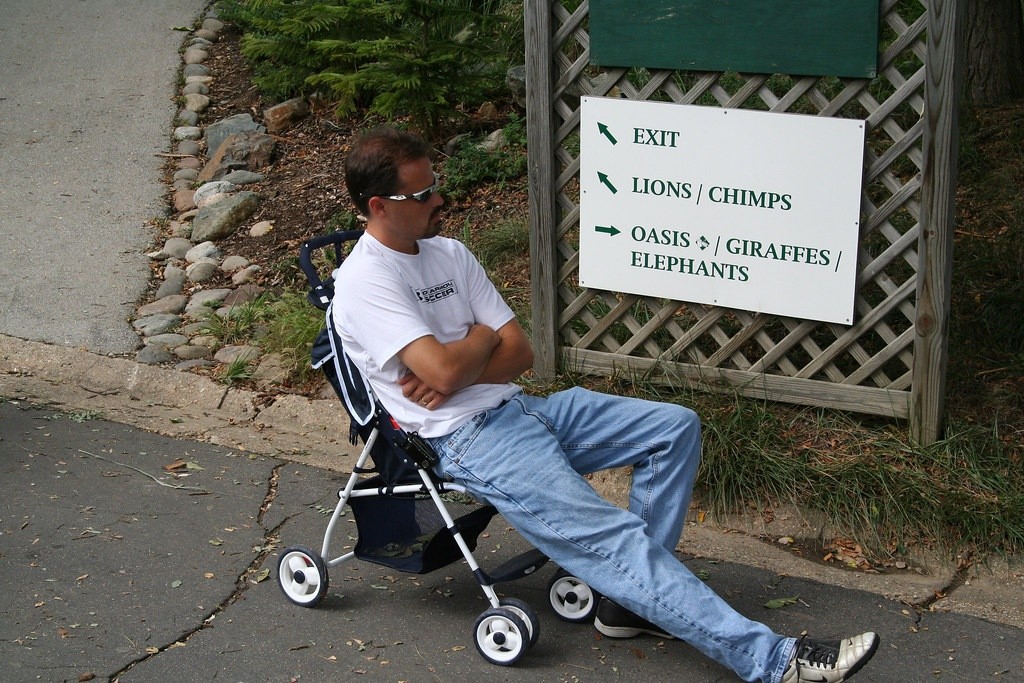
358;171;441;204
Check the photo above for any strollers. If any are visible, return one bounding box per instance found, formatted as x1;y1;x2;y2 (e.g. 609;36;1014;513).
274;228;598;667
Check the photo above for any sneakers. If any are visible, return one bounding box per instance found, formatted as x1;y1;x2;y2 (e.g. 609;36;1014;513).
780;631;880;683
594;597;685;642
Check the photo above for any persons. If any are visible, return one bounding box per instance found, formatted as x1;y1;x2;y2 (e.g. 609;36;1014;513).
331;129;879;683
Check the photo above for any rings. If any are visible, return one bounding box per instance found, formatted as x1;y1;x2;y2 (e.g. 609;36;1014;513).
421;398;427;405
